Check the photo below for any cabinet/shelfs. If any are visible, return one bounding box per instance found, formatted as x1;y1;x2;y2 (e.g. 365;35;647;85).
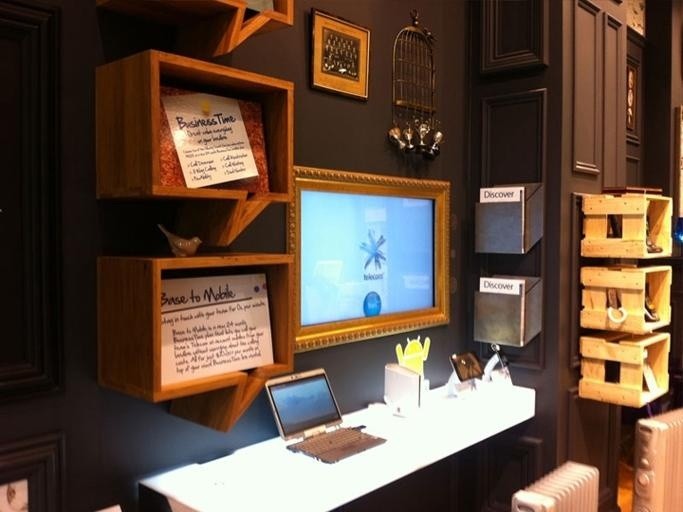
93;2;301;434
577;192;671;409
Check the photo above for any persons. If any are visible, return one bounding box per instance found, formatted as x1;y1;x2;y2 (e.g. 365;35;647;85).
322;33;358;78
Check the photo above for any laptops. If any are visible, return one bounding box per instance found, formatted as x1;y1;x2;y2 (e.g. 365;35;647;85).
264;367;387;462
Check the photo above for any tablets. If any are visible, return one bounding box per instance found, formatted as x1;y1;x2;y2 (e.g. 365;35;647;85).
448;350;485;384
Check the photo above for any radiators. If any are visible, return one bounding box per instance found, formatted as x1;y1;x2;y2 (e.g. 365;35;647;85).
510;406;681;510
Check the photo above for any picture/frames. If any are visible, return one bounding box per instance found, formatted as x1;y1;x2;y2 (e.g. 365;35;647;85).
310;8;372;102
288;163;452;355
